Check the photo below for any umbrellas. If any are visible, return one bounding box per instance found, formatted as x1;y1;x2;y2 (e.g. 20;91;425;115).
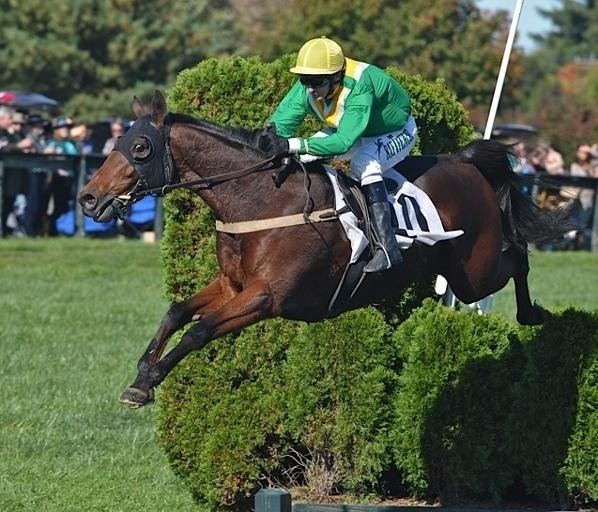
475;122;540;138
0;89;58;108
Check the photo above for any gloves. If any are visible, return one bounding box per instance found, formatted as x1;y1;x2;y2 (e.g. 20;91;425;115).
258;133;290;160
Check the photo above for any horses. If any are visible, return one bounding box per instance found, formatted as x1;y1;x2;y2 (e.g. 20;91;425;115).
76;88;584;410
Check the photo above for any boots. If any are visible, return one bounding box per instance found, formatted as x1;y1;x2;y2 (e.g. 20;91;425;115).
361;199;403;275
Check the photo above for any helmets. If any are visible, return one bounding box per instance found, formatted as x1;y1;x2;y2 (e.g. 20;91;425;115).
288;35;346;80
9;112;76;131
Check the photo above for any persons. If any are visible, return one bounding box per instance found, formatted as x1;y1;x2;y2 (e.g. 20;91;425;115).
266;36;419;276
0;106;127;236
503;140;598;251
